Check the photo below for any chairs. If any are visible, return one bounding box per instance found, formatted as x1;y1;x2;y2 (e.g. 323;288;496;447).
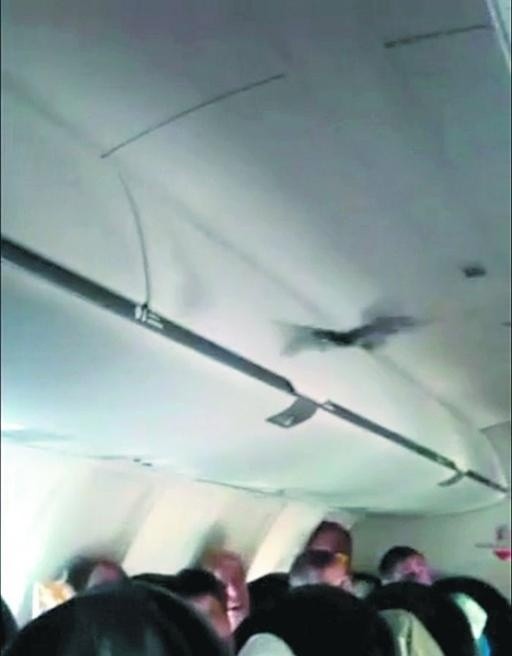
1;573;512;655
